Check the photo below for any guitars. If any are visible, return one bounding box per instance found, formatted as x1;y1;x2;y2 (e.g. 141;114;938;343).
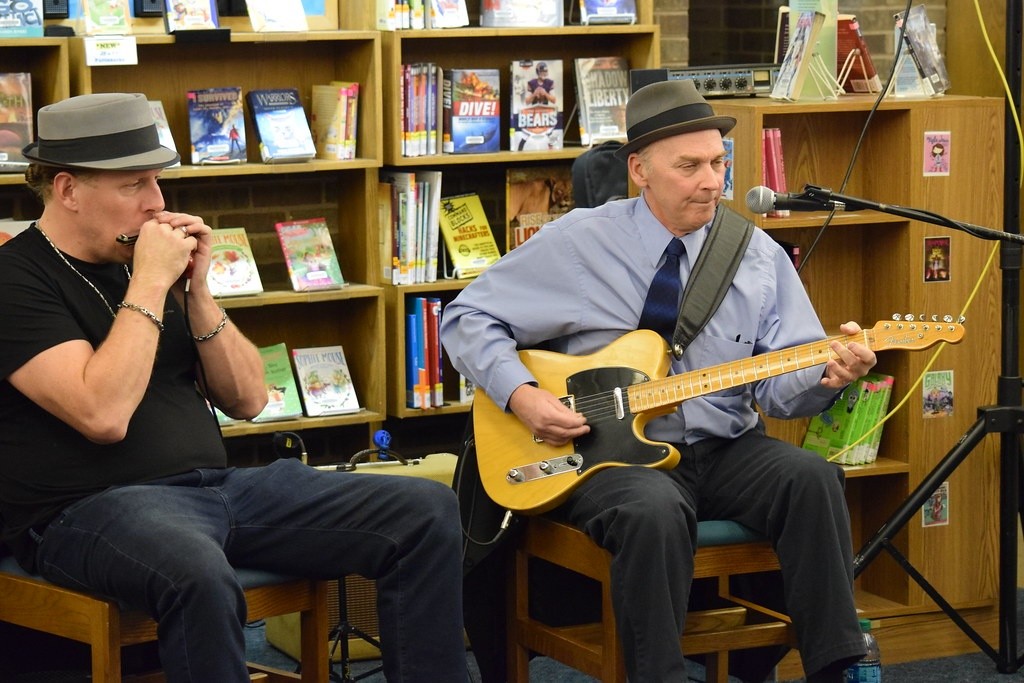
471;310;969;509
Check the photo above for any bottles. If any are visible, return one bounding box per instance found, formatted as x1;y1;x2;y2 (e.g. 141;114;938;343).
846;619;882;683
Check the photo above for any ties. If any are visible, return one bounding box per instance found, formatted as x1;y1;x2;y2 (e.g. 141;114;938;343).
636;237;685;345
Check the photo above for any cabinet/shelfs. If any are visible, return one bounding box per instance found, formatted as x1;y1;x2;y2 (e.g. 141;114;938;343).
0;0;1016;669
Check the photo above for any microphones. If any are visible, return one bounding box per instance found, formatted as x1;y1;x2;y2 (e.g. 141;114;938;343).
745;186;868;214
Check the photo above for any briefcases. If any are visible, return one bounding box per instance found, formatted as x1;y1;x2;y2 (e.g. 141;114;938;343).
266;450;458;661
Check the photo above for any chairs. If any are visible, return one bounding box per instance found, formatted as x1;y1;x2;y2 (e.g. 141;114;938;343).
0;553;330;683
506;503;806;683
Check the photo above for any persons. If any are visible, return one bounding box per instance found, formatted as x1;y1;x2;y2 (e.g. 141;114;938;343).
0;0;360;426
439;79;876;683
1;92;471;683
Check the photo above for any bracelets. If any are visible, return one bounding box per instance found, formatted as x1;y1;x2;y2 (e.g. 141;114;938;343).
117;301;164;332
192;308;227;341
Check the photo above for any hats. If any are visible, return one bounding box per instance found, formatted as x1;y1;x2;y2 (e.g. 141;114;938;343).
614;79;737;160
22;93;180;170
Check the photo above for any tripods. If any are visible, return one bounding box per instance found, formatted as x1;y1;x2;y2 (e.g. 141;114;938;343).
772;0;1024;677
294;572;385;683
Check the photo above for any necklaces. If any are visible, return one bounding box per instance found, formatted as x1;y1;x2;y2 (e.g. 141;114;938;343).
36;220;132;318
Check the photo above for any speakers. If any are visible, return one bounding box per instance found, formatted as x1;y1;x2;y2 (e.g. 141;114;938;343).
263;454;473;661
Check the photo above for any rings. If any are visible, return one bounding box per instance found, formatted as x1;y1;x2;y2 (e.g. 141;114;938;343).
178;226;188;236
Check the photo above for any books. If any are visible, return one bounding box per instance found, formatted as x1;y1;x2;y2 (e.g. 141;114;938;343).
380;0;633;409
